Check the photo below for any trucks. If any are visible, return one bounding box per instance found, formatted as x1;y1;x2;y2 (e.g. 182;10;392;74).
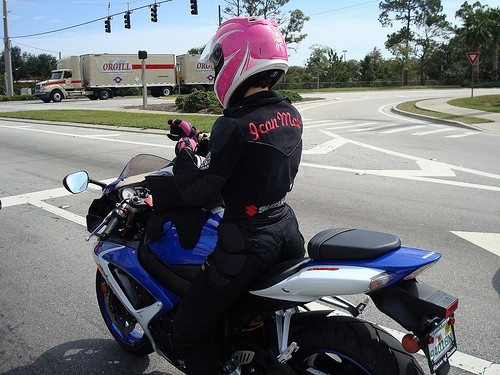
176;54;216;95
33;53;177;103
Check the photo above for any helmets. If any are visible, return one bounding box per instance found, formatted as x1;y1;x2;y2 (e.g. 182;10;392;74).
209;15;289;109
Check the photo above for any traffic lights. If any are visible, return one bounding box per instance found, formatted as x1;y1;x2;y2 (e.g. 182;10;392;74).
124;14;131;29
104;19;112;33
150;6;158;22
190;0;198;15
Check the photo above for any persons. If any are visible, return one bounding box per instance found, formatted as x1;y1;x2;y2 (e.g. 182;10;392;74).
167;16;305;375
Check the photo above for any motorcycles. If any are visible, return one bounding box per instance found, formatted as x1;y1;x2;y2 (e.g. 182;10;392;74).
62;154;461;375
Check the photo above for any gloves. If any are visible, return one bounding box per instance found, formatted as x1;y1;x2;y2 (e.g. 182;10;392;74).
168;118;200;140
175;137;198;155
